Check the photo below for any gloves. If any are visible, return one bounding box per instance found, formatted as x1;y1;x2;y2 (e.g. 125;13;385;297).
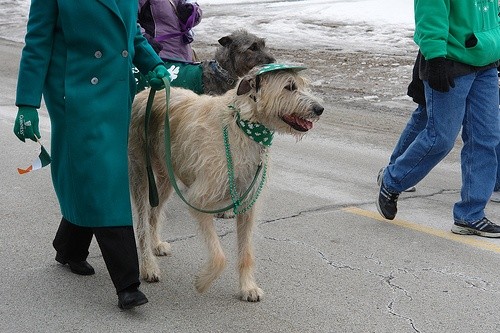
13;106;42;143
147;36;163;55
424;57;455;93
144;64;172;91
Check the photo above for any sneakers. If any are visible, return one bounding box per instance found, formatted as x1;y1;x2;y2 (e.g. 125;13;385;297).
451;216;500;238
376;167;398;221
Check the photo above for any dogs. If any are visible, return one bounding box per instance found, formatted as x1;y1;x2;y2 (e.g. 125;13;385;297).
136;27;277;97
128;60;325;303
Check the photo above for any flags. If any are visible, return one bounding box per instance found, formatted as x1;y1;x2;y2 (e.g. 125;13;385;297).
18;144;52;175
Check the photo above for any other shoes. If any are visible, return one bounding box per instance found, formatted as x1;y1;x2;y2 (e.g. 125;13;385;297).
117;287;149;310
55;252;95;275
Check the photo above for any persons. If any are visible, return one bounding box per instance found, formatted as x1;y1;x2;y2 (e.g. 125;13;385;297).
13;0;170;308
137;0;202;63
376;0;500;238
